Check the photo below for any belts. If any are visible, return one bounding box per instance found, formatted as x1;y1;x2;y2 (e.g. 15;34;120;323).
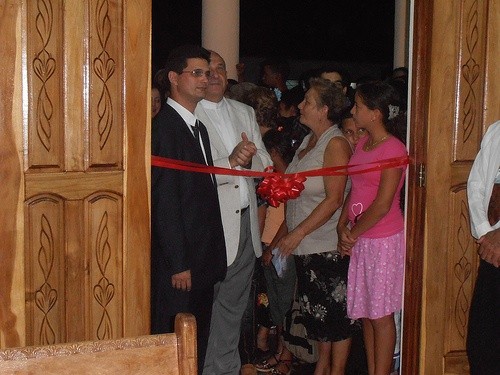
241;205;250;215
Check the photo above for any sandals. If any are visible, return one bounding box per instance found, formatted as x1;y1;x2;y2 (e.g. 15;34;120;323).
271;359;294;375
255;351;282;372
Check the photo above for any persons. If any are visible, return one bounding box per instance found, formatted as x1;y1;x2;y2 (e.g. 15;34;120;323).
467;120;500;375
255;128;297;375
278;59;409;151
150;42;227;375
274;77;353;375
336;81;407;375
195;50;274;375
150;54;284;375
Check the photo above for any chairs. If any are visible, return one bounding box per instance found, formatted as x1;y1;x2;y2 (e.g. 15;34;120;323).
0;312;197;375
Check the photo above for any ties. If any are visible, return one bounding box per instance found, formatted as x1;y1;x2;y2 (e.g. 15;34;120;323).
488;167;500;227
190;119;199;145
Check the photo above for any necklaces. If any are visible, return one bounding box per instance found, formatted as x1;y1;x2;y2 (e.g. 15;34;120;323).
363;133;393;151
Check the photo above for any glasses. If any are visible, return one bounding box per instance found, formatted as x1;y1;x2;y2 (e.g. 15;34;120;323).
180;70;210;79
152;97;162;104
